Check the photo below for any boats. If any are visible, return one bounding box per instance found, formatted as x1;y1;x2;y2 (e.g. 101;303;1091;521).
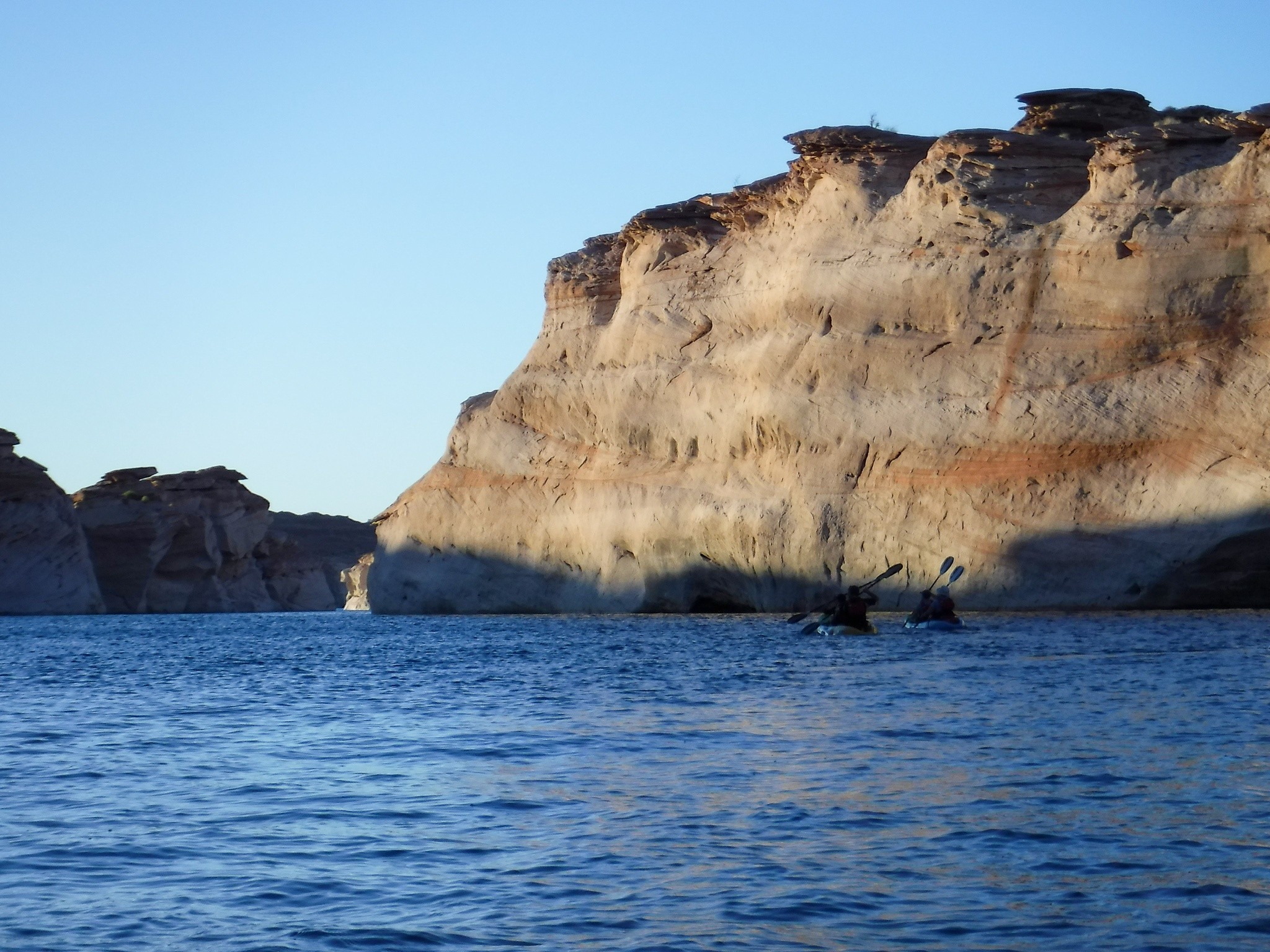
903;610;966;632
817;618;879;638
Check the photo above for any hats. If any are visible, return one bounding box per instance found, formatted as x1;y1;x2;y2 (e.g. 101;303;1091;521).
936;586;950;597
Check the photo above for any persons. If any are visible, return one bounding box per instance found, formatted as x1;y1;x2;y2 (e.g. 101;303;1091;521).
907;586;959;624
820;585;879;632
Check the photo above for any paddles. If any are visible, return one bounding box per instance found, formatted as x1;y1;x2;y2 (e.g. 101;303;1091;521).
787;562;904;635
907;557;966;629
